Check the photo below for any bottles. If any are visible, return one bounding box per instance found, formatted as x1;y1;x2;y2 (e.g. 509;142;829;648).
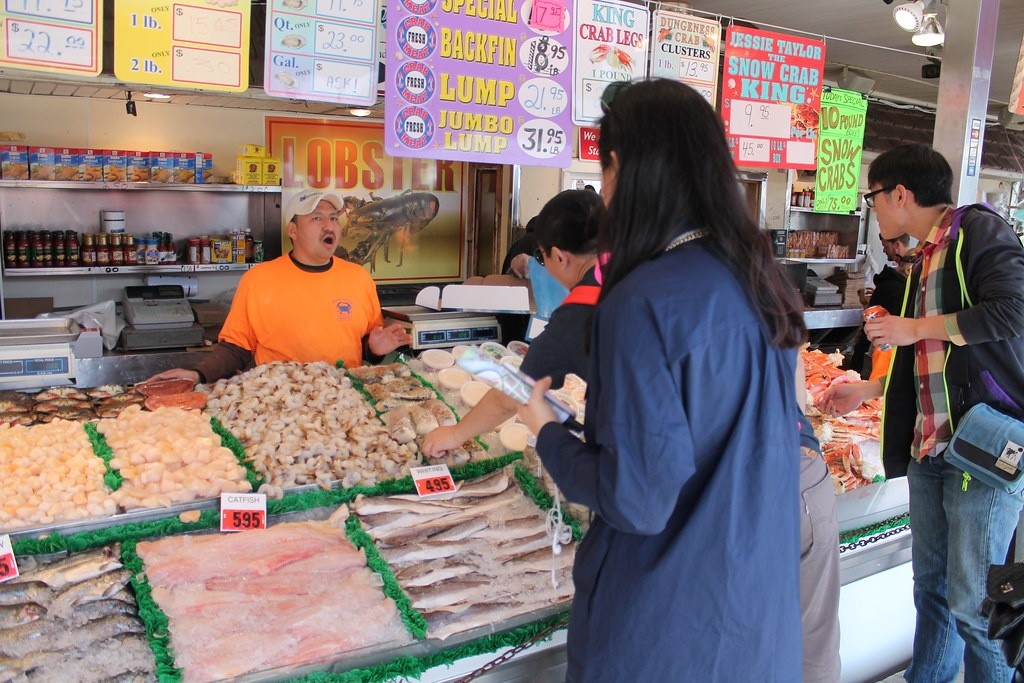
3;230;176;268
233;228;253;262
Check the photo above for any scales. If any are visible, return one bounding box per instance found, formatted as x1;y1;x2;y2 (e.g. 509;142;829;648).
382;305;504;350
1;316;81;392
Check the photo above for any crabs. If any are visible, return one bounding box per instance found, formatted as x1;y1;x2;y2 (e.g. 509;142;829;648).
800;350;885;494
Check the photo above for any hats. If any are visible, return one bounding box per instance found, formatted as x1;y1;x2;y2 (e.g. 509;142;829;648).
286;189;344;224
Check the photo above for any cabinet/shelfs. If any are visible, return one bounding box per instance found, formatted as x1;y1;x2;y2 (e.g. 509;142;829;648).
786;193;868;272
0;178;282;277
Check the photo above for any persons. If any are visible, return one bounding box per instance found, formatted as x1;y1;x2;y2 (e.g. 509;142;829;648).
420;188;846;683
515;82;805;683
145;189;413;393
495;213;541;349
811;145;1024;683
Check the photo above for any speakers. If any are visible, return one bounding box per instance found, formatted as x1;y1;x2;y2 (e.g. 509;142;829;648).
837;72;875;93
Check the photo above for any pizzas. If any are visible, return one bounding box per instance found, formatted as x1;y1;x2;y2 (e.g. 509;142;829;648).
782;101;821;130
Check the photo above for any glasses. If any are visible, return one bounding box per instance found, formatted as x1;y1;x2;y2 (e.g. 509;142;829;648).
534;242;561;266
601;81;625;122
864;184;899;208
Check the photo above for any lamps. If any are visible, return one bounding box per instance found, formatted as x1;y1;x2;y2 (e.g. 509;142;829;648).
892;0;933;32
350;108;371;116
124;90;138;118
912;13;945;47
997;105;1024;132
836;68;875;94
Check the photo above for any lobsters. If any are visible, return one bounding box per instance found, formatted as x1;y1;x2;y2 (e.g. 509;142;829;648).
332;188;439;274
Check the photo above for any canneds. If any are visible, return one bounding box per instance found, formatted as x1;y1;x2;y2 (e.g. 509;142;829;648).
861;305;898;351
186;238;211;264
253;240;265;263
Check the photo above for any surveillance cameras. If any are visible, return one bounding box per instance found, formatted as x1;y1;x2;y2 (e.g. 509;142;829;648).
922;64;940;79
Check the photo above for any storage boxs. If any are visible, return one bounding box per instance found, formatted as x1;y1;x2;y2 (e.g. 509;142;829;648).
127;151;150;181
195;152;213;183
0;144;29;180
103;149;127;182
55;148;78;181
173;152;195;183
150;151;173;183
415;274;550;343
228;143;280;185
78;148;103;181
28;146;55;180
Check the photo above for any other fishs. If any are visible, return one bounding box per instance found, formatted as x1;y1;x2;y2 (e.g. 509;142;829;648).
0;379;147;428
349;467;581;636
0;538;161;682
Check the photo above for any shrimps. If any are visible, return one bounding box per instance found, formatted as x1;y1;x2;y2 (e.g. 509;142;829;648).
199;359;422;498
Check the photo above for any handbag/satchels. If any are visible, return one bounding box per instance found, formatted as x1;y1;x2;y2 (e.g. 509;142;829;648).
985;560;1024;675
944;403;1023;495
